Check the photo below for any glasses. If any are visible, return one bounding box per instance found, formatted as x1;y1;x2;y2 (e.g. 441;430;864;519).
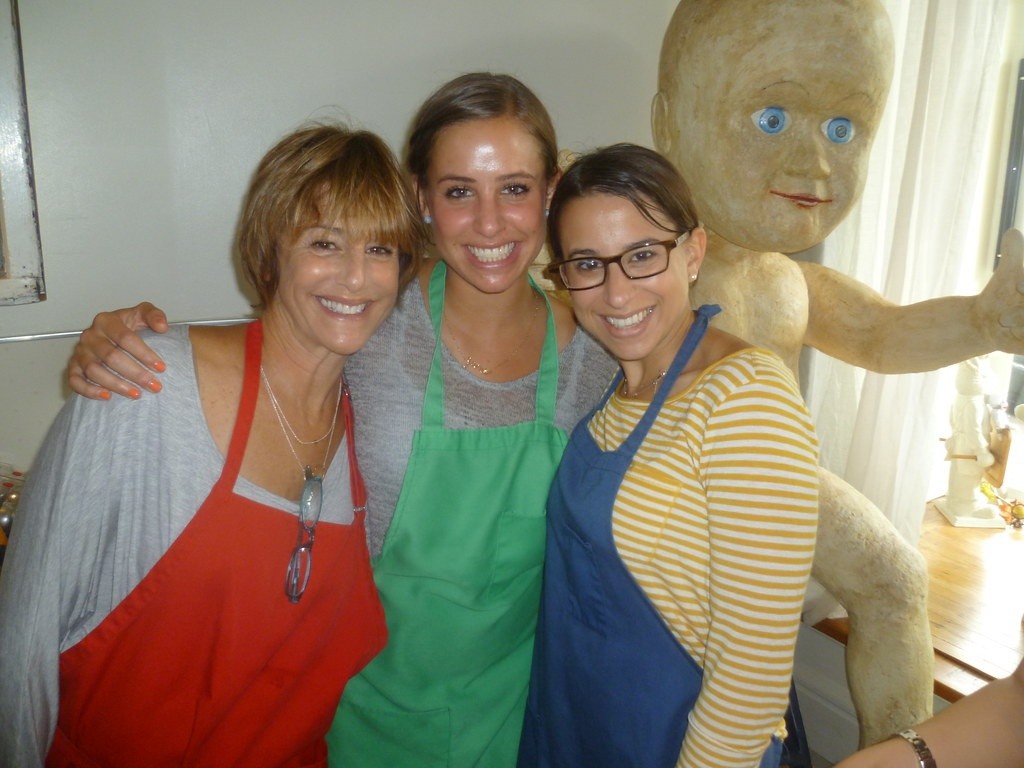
547;231;690;292
285;465;323;603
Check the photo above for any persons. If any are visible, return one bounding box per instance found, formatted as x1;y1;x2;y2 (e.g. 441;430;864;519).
517;143;818;767
68;73;618;768
554;0;1023;750
0;125;424;768
832;661;1024;768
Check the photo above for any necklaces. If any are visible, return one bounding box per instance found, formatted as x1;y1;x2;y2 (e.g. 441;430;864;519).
260;365;342;479
623;370;668;398
446;288;539;374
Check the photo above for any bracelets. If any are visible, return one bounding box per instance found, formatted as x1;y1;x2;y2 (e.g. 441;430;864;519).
892;728;936;768
